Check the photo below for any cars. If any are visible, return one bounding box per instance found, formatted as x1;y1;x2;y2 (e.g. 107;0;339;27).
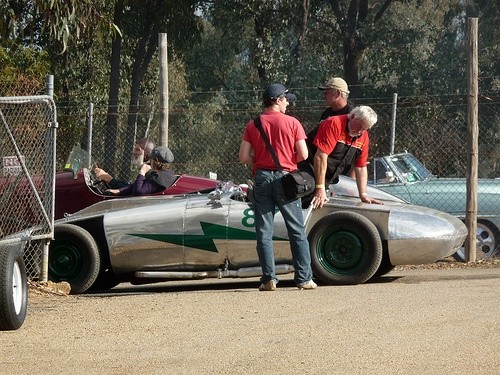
364;152;500;261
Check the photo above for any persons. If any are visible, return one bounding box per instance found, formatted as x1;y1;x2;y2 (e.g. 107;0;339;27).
297;105;385;211
319;77;358;122
239;83;319;291
94;139;178;197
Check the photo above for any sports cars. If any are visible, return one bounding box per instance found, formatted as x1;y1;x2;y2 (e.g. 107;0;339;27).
10;174;468;284
1;167;250;239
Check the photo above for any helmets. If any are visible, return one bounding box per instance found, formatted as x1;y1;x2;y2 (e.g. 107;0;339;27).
135;135;156;160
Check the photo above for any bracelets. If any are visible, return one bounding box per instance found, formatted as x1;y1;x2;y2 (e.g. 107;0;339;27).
316;184;325;189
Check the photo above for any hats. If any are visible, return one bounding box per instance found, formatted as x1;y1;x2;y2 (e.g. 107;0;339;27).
263;82;289;99
152;145;174;163
318;77;349;94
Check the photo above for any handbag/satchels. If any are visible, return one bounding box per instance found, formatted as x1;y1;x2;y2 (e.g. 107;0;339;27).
285;171;315;199
